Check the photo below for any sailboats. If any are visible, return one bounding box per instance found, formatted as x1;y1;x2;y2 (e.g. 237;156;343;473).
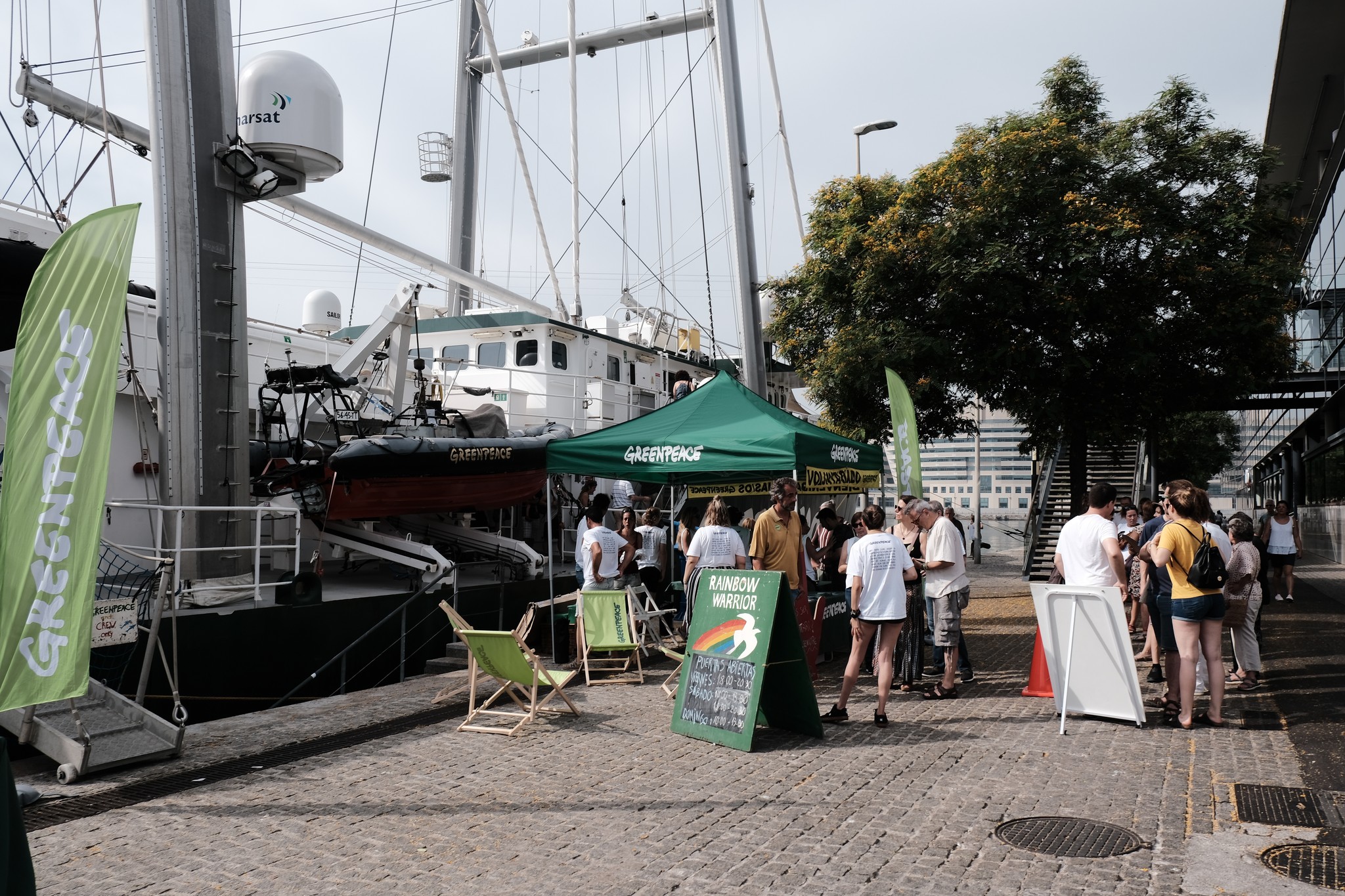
0;0;770;732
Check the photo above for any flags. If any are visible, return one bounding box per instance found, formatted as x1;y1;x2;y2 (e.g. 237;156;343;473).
0;202;141;715
884;365;924;502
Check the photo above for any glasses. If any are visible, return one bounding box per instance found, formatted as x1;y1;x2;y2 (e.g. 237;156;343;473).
911;507;927;525
1266;504;1274;506
1120;502;1131;506
1277;506;1288;509
1123;505;1135;509
853;522;863;528
623;508;635;513
895;505;905;512
1165;503;1173;509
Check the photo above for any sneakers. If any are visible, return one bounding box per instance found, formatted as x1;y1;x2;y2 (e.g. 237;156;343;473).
922;666;945;677
602;657;622;668
561;657;585;670
960;669;974;682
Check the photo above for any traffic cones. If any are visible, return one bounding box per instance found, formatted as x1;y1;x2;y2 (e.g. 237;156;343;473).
1021;623;1055;698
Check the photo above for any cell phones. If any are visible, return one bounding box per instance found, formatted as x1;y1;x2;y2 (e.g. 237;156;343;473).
1117;531;1126;536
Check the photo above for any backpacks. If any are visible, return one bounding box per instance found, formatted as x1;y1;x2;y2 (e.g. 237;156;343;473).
1169;522;1228;588
1047;556;1065;585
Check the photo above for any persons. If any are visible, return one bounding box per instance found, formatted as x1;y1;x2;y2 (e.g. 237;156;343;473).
1054;479;1303;731
673;369;695;402
541;473;984;728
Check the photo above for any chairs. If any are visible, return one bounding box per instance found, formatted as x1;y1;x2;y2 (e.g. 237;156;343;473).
438;597;582;741
573;575;687;700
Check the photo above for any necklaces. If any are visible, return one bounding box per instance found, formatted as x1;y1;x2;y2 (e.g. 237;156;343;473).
900;522;915;540
869;529;881;533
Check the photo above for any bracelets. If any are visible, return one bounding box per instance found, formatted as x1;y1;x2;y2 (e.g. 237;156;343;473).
683;581;688;585
925;562;930;571
920;563;925;570
923;563;927;571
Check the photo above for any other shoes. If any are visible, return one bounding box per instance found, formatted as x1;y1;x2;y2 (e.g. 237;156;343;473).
1147;665;1163;683
1275;594;1284;601
1286;594;1294;602
676;626;688;641
820;703;848;722
559;558;572;563
873;708;889;727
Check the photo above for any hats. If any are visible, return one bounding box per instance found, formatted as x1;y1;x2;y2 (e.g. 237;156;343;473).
1152;500;1163;507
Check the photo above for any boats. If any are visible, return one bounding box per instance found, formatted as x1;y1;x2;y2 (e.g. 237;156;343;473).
248;422;576;524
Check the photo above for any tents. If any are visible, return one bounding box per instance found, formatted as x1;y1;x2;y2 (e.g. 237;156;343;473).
542;368;886;664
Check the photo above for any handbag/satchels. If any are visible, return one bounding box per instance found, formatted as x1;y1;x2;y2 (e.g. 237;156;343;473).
1222;599;1248;629
543;501;558;521
1125;553;1136;573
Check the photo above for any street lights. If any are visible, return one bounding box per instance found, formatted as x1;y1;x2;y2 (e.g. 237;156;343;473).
854;118;894;513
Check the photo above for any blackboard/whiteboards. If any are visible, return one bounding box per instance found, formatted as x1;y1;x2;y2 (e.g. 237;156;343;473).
669;570;826;755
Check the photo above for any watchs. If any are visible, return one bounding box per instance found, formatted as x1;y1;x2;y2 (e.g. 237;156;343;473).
851;610;860;619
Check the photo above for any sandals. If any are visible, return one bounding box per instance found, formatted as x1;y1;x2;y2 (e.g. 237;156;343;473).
1134;652;1152;662
1228;668;1236;673
1224;673;1246;684
1163;699;1180;719
900;680;913;691
1151;693;1181;708
1237;678;1260;690
1128;624;1135;634
920;681;958;700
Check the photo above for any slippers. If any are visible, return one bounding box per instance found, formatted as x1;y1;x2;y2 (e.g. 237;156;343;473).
1191;711;1225;728
1162;714;1195;729
